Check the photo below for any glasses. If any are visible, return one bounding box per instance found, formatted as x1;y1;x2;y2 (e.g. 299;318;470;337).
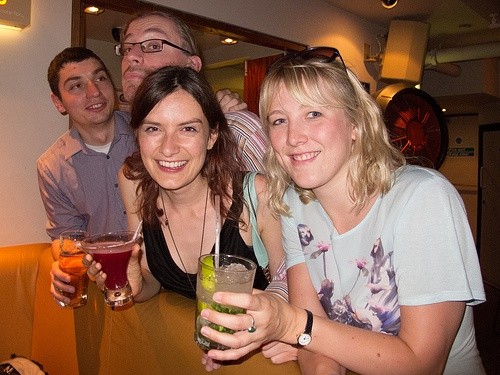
268;46;346;69
114;38;194;56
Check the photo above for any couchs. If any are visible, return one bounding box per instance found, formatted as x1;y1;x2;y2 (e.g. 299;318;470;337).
0;243;302;375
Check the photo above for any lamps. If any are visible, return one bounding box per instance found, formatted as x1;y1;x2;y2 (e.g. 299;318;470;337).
84;4;105;16
220;35;238;46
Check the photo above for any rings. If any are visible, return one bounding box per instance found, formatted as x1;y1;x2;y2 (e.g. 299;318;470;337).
242;314;256;333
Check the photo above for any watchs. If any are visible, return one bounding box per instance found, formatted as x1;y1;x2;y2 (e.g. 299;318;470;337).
291;309;313;348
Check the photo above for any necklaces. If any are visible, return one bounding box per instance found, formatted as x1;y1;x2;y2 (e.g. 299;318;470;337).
160;167;211;294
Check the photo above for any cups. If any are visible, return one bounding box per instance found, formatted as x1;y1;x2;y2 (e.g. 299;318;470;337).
58;230;89;308
194;254;257;350
78;231;141;310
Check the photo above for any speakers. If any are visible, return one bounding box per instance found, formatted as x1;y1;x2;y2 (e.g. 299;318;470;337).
380;19;430;84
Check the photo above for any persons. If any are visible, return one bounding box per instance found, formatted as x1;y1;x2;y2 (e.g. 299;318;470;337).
100;8;299;365
200;45;488;375
35;47;249;309
82;65;283;300
